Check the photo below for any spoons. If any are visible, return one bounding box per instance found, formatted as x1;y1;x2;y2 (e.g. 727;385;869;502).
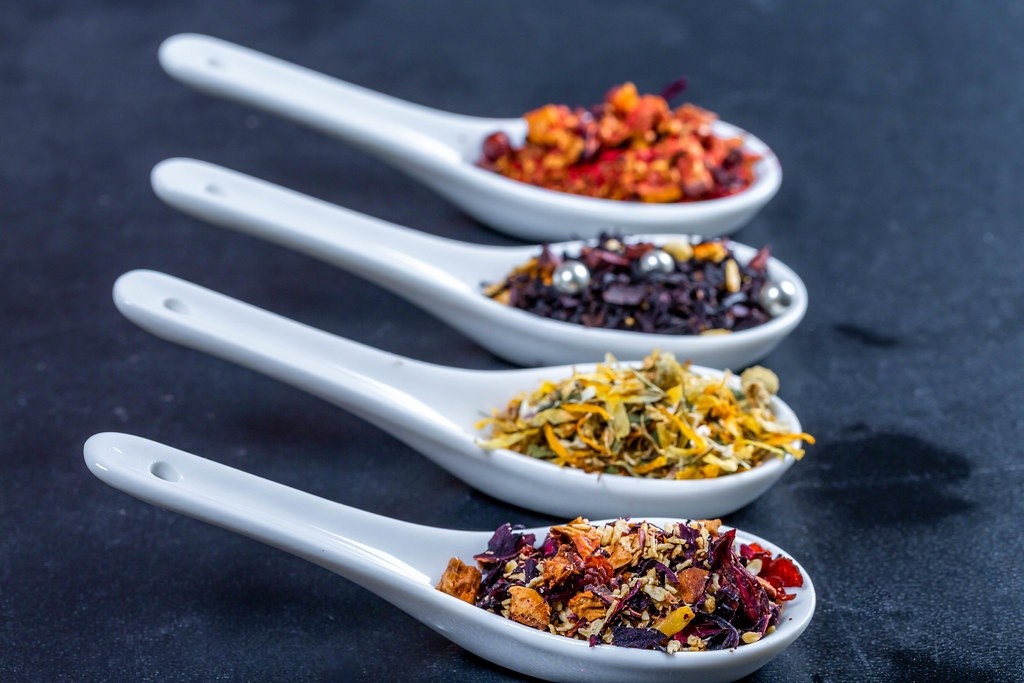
158;33;781;241
113;268;804;518
83;430;816;683
151;158;810;374
